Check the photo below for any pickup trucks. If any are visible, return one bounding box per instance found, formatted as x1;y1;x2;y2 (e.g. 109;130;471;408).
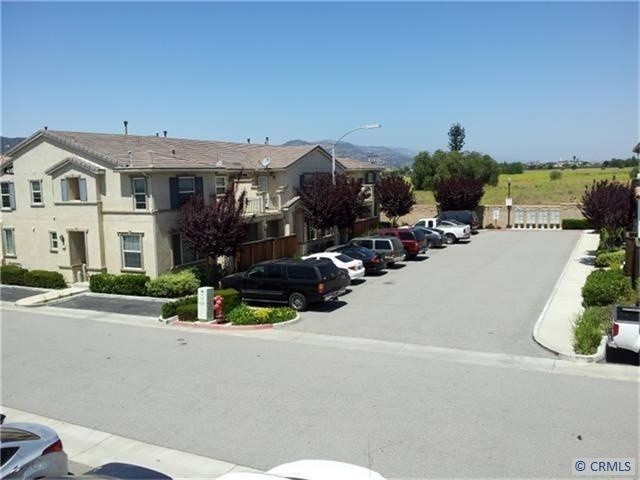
605;304;640;364
412;218;471;245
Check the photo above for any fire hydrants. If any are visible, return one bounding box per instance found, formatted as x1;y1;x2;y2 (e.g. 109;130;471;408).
212;295;226;325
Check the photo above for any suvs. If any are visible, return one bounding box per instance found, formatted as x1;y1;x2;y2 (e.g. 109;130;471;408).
221;258;352;312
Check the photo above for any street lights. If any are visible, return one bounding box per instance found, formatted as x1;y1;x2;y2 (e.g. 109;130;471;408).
506;178;512;229
330;121;382;246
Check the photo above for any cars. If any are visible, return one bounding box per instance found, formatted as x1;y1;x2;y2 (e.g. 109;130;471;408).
301;252;365;285
415;226;446;249
324;245;387;274
0;413;392;480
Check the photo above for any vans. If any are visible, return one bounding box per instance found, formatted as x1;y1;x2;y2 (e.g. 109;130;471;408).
346;236;407;268
433;210;481;230
380;228;427;260
